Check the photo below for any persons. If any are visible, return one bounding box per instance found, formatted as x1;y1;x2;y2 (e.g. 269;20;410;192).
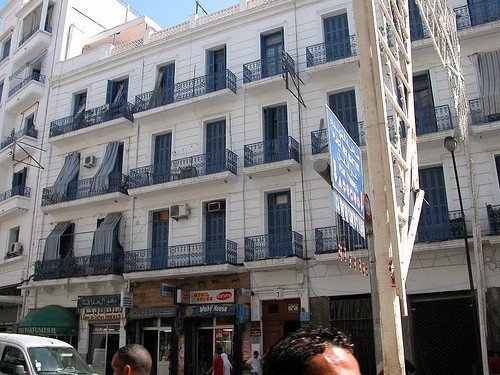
259;323;362;375
246;349;262;375
111;344;153;375
220;353;233;375
205;350;224;375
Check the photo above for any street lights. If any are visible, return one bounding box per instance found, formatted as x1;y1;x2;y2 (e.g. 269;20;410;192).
443;135;483;375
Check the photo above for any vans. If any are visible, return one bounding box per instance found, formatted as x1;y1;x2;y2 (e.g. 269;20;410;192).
0;333;98;375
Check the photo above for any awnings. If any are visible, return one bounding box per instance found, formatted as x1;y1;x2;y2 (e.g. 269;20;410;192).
127;306;182;322
19;305;79;336
183;304;240;322
